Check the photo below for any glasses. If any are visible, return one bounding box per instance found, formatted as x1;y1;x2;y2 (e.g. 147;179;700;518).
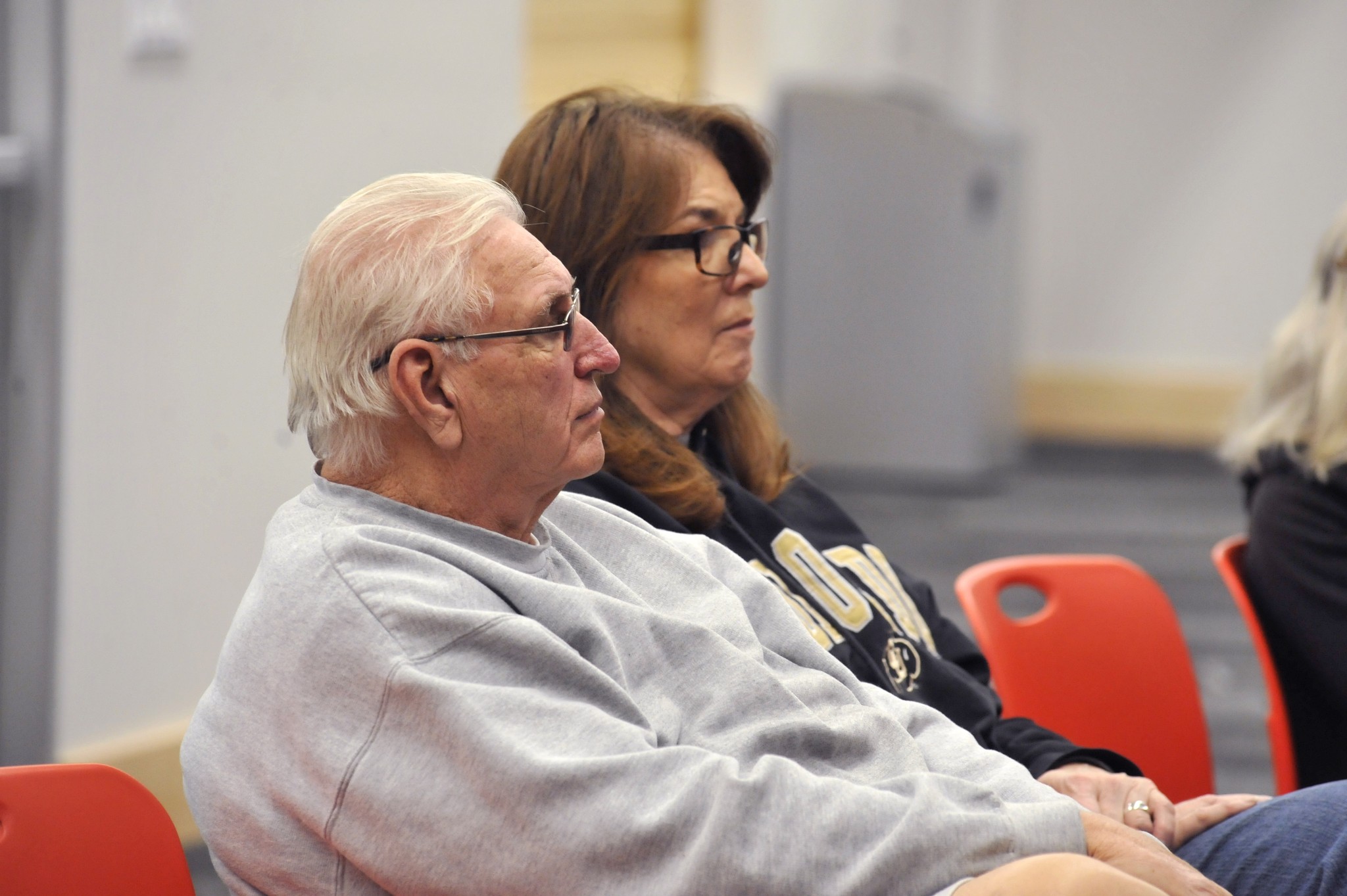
634;218;770;278
371;287;581;371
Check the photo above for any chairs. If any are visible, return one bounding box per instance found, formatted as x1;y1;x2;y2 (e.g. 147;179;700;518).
1211;531;1295;795
956;555;1215;803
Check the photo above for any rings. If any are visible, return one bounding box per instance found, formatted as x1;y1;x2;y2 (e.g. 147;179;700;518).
1126;798;1151;815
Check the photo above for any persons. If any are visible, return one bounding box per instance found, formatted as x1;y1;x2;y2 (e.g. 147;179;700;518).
1231;207;1346;793
176;171;1245;896
488;85;1347;896
